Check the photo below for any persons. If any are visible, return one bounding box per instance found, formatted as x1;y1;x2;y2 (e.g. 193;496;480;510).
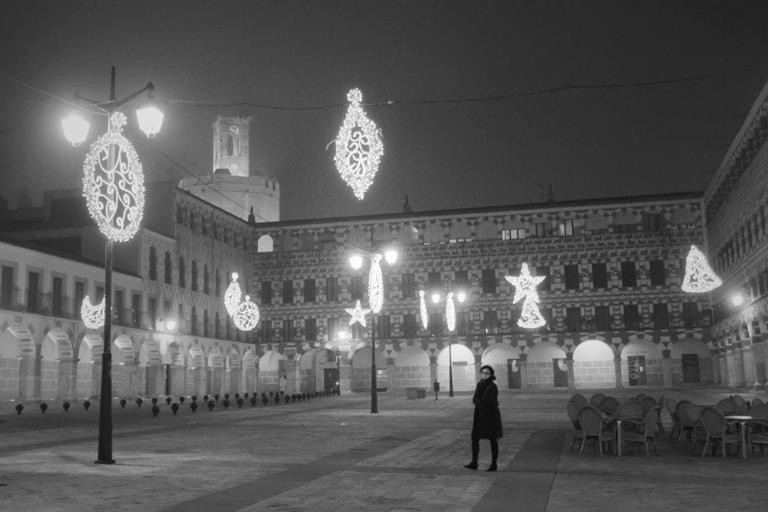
463;365;503;472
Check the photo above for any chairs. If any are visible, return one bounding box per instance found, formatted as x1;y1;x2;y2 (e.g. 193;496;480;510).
567;392;768;460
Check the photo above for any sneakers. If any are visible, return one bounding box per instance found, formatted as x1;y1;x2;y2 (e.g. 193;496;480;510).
463;461;478;470
485;462;498;472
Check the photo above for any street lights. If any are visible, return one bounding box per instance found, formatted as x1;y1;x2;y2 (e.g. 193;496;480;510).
67;65;154;462
434;277;466;397
352;223;398;415
